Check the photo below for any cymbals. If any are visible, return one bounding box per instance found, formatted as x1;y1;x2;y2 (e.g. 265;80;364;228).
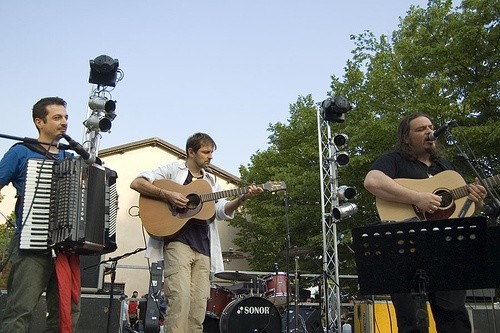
222;251;248;259
214;272;258;280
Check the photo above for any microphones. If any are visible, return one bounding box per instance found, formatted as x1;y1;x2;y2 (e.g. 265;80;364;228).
62;133;90;160
428;120;454;141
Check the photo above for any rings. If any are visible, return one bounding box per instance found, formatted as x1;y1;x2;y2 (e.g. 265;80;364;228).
432;206;437;211
429;209;433;214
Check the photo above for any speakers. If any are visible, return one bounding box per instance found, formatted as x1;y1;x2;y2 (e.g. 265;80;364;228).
0;253;125;333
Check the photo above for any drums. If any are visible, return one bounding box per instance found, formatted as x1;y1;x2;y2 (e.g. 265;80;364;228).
206;284;235;320
219;295;282;333
262;271;294;305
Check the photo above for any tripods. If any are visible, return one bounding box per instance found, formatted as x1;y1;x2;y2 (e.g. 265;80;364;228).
289;254;308;333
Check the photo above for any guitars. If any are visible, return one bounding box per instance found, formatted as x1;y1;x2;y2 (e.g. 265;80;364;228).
375;169;500;222
138;179;287;241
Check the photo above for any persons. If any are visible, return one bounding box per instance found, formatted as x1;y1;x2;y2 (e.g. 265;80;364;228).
129;132;264;333
348;299;356;304
121;291;148;333
0;96;75;333
305;297;312;304
363;113;487;333
